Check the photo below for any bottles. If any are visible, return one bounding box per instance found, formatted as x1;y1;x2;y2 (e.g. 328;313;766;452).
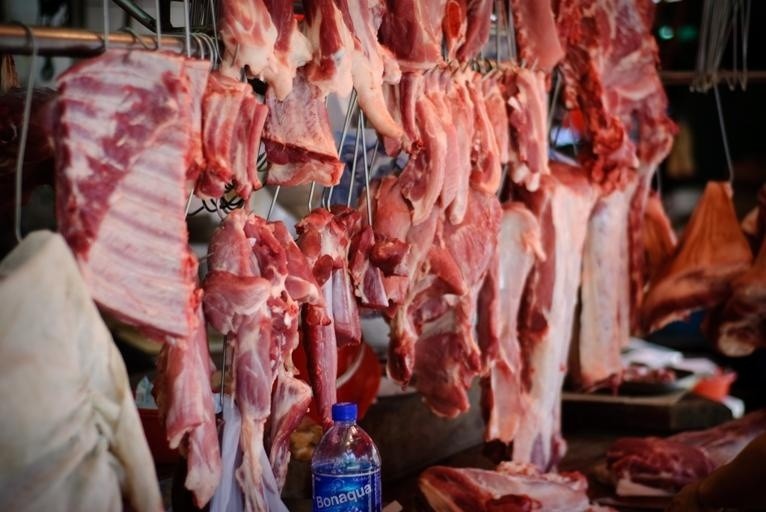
309;400;383;512
